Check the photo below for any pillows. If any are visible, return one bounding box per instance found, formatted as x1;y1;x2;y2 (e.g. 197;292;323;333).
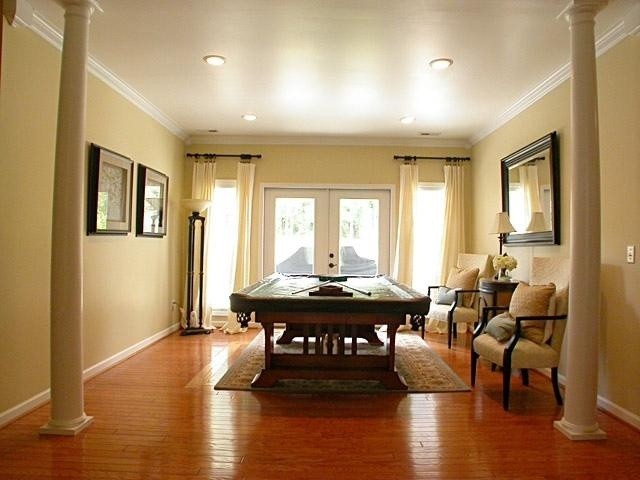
483;281;556;342
434;265;481;306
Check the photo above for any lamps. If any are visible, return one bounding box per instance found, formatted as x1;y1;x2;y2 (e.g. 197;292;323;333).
488;212;517;254
524;210;548;232
179;197;211;334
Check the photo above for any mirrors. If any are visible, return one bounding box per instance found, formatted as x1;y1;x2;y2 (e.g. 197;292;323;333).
499;130;562;247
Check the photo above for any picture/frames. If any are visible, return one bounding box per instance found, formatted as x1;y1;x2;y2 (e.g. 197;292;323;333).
86;141;169;238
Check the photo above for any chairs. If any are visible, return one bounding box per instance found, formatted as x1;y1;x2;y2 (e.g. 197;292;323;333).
421;252;493;349
470;284;567;410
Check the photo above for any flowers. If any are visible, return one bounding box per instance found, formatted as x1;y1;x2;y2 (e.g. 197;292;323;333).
493;253;518;278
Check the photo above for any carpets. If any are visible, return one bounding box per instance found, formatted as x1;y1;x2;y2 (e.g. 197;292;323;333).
213;323;472;393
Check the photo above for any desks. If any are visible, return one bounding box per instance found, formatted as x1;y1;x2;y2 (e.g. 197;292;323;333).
472;280;519;332
229;269;432;390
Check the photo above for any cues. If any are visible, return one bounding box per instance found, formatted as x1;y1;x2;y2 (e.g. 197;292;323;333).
332;279;371;296
292;279;333;295
